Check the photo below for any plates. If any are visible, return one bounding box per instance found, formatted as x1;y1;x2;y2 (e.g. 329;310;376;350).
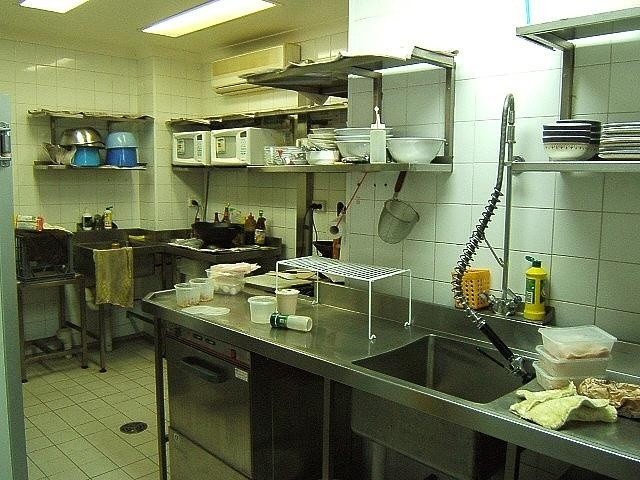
597;122;639;161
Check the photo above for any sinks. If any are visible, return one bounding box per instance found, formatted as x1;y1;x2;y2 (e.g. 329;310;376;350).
346;332;539;480
79;239;147;250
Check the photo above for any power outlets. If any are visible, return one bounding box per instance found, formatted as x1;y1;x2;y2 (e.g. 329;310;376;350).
189;199;198;206
313;199;326;212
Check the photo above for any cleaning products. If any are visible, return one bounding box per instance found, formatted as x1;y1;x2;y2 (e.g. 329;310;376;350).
81;207;93;231
102;205;114;230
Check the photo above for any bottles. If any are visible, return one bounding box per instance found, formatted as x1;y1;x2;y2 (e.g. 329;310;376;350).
221;206;231;223
243;212;256;243
82;210;94;231
94;210;103;231
370;113;386;165
255;211;266;244
213;212;220;223
268;312;314;332
103;208;113;228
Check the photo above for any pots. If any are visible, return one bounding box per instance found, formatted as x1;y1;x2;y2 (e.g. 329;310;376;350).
191;219;243;250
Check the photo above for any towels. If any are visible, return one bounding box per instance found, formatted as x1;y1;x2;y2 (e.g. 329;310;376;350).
92;246;134;310
510;375;640;430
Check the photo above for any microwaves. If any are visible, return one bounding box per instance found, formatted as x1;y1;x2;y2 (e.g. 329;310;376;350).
210;127;283;167
172;131;211;167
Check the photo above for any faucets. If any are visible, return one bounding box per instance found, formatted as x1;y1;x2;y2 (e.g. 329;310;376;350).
451;94;533;382
92;211;109;230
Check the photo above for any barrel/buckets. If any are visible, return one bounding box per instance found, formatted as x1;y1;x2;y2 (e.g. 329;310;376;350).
104;148;138;168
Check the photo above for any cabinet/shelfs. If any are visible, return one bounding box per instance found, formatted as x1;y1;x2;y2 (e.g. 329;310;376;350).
241;49;455;172
30;108;148;173
509;7;640;174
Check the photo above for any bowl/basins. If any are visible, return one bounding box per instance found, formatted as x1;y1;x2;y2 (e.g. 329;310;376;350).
335;125;393;163
387;135;446;166
106;130;140;148
304;127;339;165
542;115;601;162
57;127;102;146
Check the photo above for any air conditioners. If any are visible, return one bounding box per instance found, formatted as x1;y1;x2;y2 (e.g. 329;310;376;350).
211;43;301;98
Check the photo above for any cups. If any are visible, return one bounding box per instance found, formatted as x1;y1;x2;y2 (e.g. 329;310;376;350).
248;295;277;323
175;277;215;307
276;288;299;314
